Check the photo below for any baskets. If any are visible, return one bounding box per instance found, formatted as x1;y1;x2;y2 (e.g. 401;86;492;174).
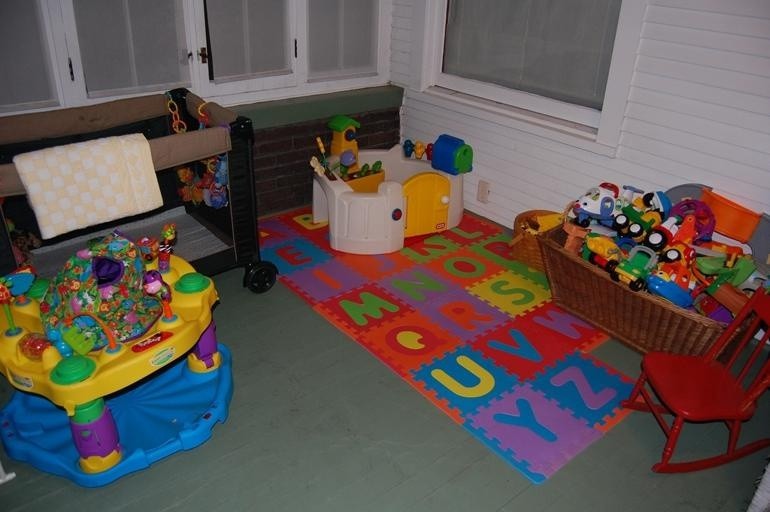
509;210;739;359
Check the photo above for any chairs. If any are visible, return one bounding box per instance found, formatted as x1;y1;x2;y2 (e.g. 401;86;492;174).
620;289;770;472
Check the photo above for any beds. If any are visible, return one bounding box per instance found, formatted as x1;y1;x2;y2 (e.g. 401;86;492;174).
0;88;279;295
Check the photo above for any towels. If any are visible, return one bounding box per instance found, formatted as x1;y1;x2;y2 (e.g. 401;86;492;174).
12;132;164;241
